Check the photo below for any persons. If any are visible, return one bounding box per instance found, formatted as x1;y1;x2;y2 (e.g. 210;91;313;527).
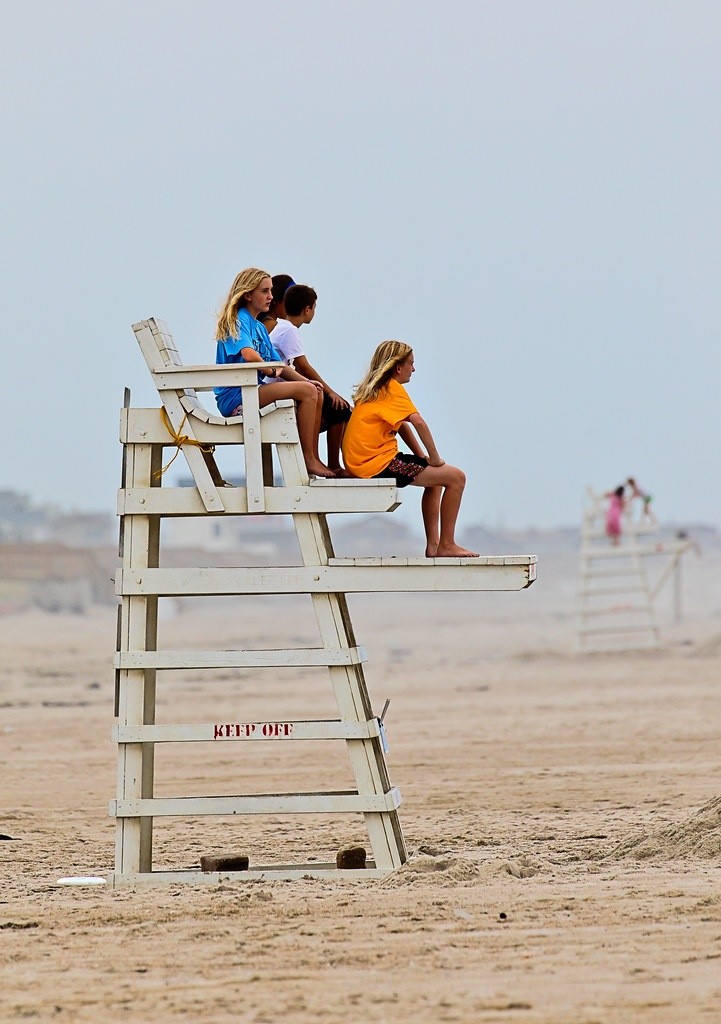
606;486;625;545
342;340;480;557
257;274;352;477
213;268;335;477
625;479;651;514
